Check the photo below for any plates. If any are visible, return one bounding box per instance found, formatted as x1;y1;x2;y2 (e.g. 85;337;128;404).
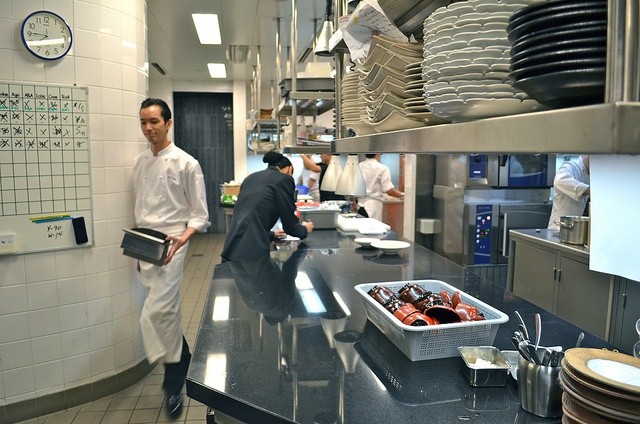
341;72;379;135
404;62;451;123
560;347;640;424
355;237;378;247
371;239;410;255
422;0;544;122
507;0;607;109
355;34;427;129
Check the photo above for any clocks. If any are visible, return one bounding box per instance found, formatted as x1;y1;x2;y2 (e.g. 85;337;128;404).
21;10;72;60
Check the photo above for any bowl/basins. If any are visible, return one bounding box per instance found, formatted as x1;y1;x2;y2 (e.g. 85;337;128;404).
368;283;486;326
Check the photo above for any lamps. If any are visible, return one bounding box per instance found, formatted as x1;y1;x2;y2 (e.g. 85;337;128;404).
320;155;343;192
313;14;335;57
335;155;367;196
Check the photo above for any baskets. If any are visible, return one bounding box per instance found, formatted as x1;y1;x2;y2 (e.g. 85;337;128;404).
353;279;511;362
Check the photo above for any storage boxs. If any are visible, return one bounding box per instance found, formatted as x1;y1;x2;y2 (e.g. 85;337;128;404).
224;180;240;196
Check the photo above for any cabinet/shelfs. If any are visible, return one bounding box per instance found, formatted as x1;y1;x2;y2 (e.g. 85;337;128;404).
271;17;333;154
609;271;640;356
247;45;288;155
509;228;614;341
334;0;640;154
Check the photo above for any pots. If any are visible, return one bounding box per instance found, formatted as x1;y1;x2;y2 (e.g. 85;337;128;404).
560;214;590;245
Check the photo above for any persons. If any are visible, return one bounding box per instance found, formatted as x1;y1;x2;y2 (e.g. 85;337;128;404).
131;97;211;417
547;153;589;230
357;151;404;221
301;154;344;200
297;152;321;200
220;149;315;261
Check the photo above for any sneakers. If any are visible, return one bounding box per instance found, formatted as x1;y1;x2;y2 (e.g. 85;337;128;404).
167;391;184;418
